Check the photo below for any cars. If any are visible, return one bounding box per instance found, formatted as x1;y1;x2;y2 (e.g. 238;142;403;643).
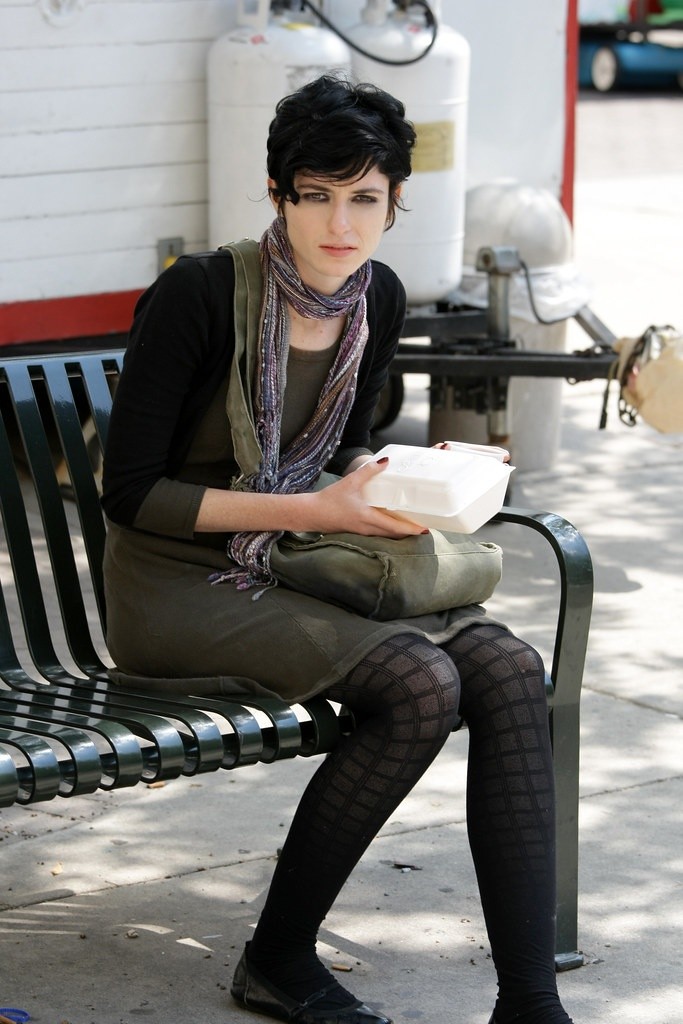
576;16;683;94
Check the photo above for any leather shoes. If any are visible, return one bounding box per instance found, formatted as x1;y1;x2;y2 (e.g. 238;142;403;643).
230;939;393;1024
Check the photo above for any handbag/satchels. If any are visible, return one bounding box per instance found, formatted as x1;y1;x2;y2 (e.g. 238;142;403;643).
270;527;502;623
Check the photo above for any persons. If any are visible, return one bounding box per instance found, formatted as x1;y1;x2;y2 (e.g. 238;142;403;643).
101;74;575;1024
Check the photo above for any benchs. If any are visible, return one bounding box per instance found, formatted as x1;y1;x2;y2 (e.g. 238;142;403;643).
0;347;595;971
